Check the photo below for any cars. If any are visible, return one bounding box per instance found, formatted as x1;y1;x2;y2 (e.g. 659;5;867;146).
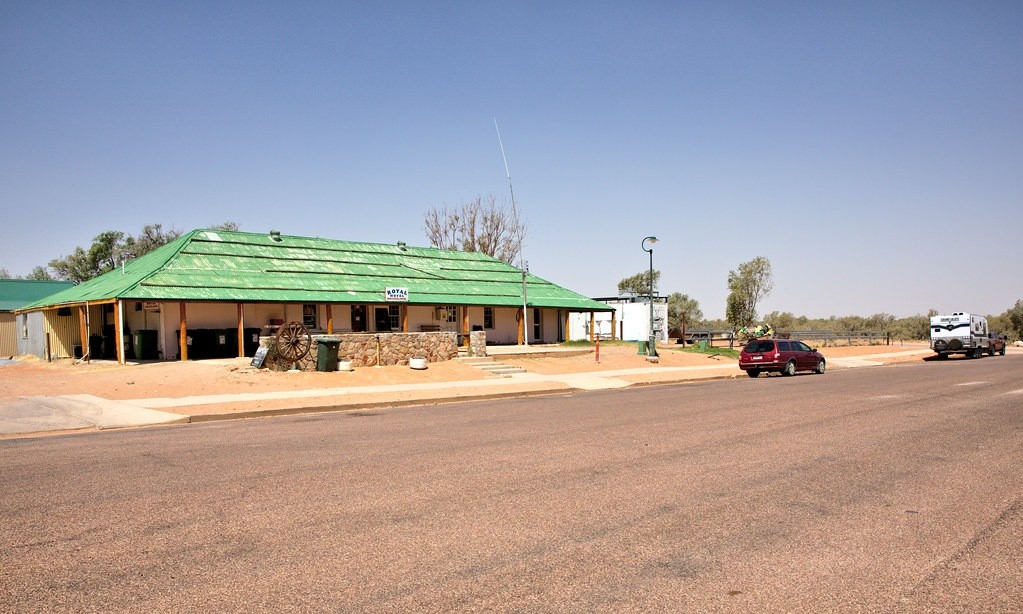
738;338;826;377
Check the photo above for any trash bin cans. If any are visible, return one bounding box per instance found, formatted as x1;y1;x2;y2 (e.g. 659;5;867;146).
134;330;159;360
316;338;343;371
176;328;262;360
638;341;647;355
698;338;707;352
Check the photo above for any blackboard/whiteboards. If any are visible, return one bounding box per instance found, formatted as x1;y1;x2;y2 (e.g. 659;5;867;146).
250;346;269;369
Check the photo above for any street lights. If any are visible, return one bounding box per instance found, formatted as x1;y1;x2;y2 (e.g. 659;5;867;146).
641;235;660;365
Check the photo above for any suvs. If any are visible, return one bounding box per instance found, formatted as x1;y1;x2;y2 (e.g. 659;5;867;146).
988;330;1006;356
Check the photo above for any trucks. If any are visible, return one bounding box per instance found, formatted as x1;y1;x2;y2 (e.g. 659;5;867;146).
929;312;989;359
